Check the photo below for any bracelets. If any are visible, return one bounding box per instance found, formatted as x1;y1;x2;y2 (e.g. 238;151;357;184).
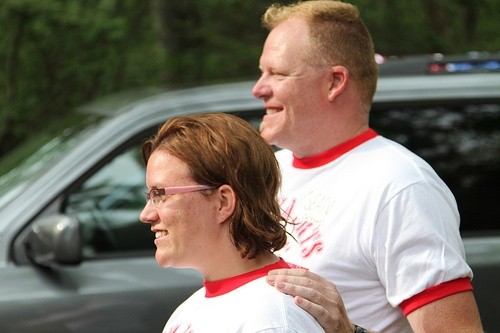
354;323;365;333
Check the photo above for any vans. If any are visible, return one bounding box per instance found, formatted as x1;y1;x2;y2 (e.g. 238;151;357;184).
0;73;500;333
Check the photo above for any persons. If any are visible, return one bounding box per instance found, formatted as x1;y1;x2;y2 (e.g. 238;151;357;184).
138;110;327;332
252;0;486;333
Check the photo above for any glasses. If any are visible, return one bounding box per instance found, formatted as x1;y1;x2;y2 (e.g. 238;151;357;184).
144;184;216;208
